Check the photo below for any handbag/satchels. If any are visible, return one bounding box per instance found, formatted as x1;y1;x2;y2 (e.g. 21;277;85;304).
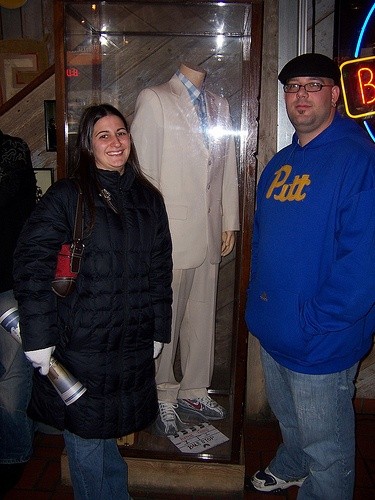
51;173;88;298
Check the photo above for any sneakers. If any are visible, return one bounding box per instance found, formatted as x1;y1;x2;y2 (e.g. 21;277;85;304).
157;399;182;438
251;464;308;492
186;397;227;421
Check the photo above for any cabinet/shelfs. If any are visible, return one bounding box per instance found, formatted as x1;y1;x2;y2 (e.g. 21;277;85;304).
53;0;264;500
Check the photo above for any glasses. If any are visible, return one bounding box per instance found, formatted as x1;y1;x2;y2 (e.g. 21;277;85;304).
283;83;332;93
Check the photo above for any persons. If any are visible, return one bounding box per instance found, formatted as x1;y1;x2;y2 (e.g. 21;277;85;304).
245;53;375;500
13;103;172;500
130;64;240;434
0;130;35;499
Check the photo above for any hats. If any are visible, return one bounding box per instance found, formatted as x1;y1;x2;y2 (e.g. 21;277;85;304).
278;53;342;84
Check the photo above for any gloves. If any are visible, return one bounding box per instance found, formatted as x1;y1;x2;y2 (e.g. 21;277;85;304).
153;340;164;360
24;346;57;376
